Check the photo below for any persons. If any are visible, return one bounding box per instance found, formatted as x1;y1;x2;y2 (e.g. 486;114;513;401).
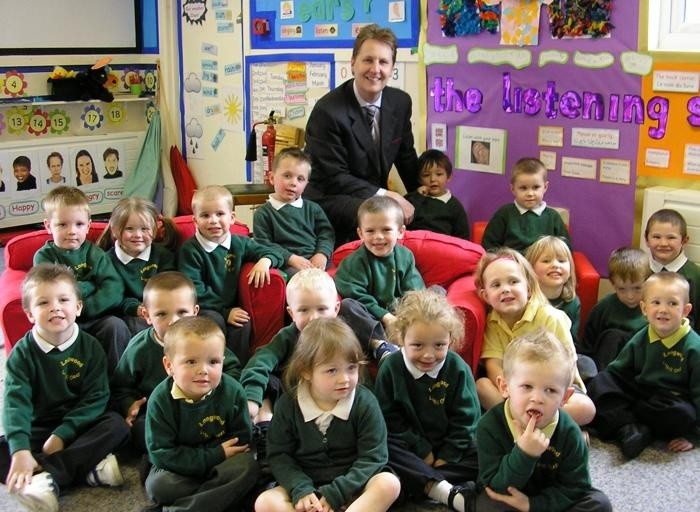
582;267;700;460
471;141;489;166
235;266;376;459
44;151;67;187
296;21;421;255
470;243;598;453
0;166;7;193
74;150;99;187
251;145;339;285
12;155;37;192
525;231;599;392
0;260;133;511
93;194;178;341
101;146;123;180
32;183;134;379
372;285;484;511
642;211;700;339
249;313;406;511
142;313;262;512
332;193;427;370
573;244;653;373
467;326;615;512
176;183;287;369
399;148;473;247
106;268;244;462
479;155;571;256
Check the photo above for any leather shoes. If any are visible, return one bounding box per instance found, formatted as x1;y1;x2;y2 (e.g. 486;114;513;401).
447;480;480;512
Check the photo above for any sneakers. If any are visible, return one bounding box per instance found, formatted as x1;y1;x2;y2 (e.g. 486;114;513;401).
12;472;63;512
85;451;128;488
375;339;400;368
618;419;651;461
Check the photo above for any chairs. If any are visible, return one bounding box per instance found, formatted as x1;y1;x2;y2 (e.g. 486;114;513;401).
326;229;490;382
470;218;603;338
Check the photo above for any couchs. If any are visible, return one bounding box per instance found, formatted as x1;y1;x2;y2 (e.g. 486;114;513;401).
1;215;287;365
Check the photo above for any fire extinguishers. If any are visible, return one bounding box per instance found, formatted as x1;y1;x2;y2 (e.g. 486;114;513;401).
245;110;277;184
